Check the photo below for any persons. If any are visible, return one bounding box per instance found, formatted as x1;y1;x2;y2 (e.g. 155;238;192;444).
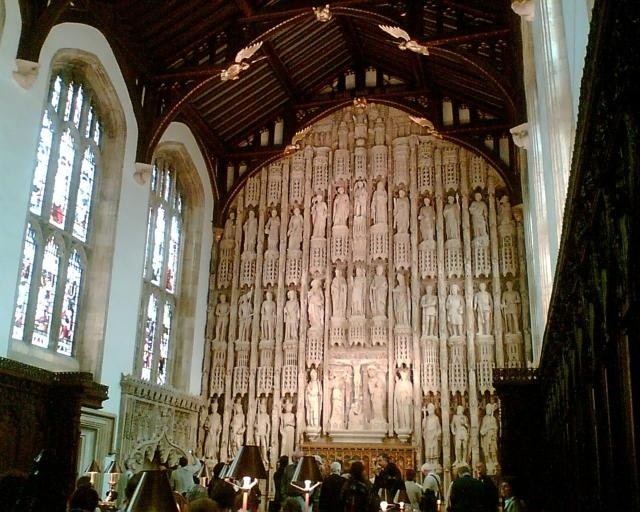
202;100;523;479
0;448;521;511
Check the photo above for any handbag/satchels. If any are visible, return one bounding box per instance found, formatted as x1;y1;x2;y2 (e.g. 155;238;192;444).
419;489;438;512
441;495;444;501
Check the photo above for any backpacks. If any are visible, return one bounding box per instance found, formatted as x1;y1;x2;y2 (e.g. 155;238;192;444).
344;479;372;511
